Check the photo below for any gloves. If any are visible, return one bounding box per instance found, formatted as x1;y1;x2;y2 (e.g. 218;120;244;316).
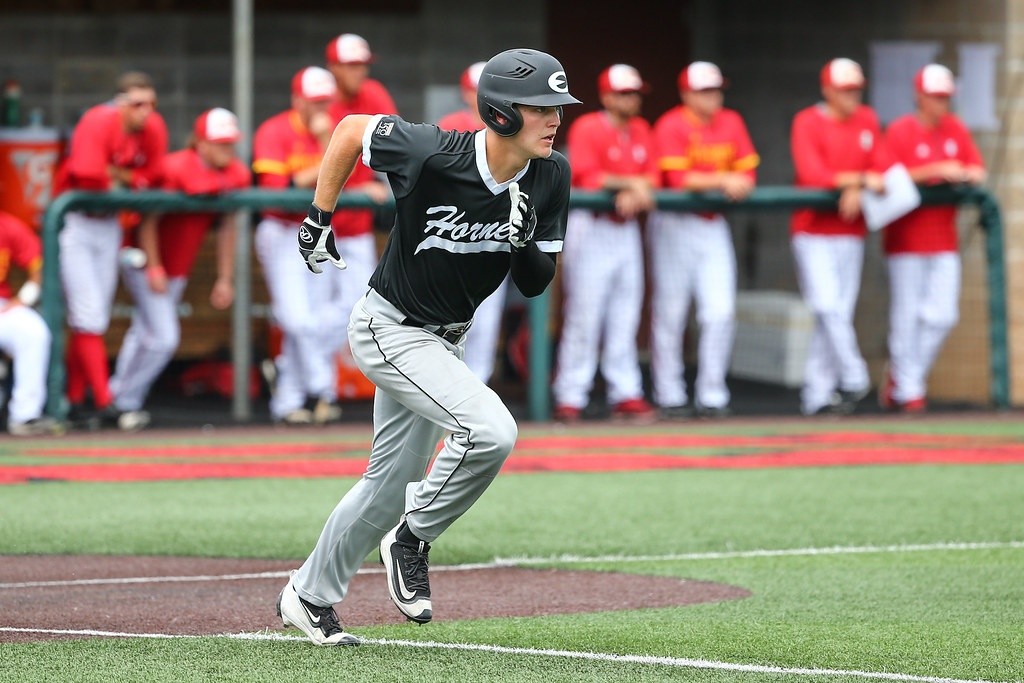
296;202;346;274
508;181;537;250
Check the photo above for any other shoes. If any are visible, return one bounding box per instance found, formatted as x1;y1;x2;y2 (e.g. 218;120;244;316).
112;409;150;433
700;406;729;419
880;366;926;415
662;406;691;420
612;399;653;418
819;387;872;417
285;408;313;425
556;406;578;423
8;417;67;439
315;401;339;424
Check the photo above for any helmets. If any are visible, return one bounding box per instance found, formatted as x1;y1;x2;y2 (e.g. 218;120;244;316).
476;48;583;137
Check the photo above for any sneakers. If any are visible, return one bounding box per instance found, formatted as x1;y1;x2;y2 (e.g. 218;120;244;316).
377;514;432;625
275;579;360;646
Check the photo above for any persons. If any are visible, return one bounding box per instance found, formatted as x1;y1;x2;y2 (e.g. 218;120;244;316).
108;109;250;414
551;59;658;423
252;32;402;424
436;61;508;388
652;61;762;417
788;54;884;420
54;73;170;432
870;64;990;414
0;211;64;438
274;43;573;652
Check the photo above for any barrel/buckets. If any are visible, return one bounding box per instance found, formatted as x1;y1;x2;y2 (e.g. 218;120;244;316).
0;125;62;232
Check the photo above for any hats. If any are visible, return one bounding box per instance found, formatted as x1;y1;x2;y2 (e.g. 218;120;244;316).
325;32;373;66
292;66;340;100
461;62;487;94
916;62;955;95
195;107;241;144
678;61;725;93
821;56;867;91
598;63;645;94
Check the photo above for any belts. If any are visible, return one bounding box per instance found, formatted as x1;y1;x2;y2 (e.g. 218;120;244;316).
695;211;716;220
366;287;472;344
592;208;628;222
